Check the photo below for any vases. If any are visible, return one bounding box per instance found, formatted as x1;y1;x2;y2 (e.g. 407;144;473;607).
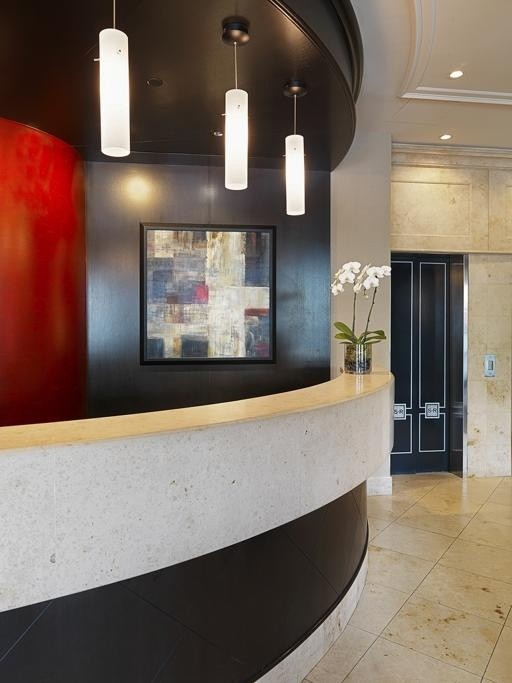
343;344;374;375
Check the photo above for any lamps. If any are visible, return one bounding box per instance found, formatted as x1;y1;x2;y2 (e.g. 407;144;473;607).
221;21;251;190
284;82;305;216
95;2;135;156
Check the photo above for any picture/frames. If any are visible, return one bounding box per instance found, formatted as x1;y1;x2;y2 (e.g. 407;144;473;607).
138;221;279;367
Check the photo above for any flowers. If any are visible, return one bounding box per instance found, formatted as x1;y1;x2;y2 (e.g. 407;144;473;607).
328;257;394;346
